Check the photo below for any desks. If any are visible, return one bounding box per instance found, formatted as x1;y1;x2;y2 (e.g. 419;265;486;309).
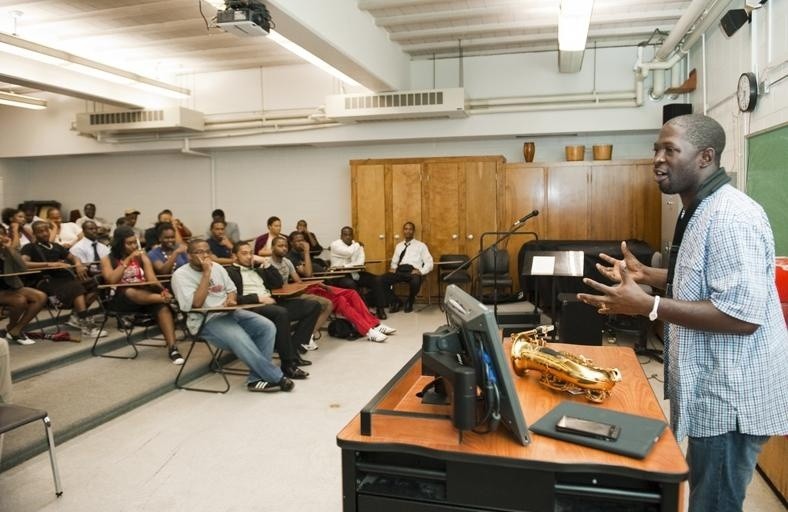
557;292;603;346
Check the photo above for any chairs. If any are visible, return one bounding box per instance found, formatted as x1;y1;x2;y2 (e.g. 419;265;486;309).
168;276;253;393
1;245;390;358
437;254;473;311
611;251;666;364
477;249;515;301
0;337;63;503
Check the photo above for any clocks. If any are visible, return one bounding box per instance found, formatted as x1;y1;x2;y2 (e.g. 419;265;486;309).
735;71;760;115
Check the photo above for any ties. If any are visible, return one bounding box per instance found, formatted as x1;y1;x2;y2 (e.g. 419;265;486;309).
397;243;411;267
91;243;100;261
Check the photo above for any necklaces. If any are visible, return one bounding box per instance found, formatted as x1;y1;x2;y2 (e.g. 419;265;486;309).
680;208;686;219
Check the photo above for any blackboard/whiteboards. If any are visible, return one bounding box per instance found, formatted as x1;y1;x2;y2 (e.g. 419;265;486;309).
743;121;788;259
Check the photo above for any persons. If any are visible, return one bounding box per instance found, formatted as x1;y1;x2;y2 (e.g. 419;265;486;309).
287;230;396;342
330;227;387;320
577;114;788;512
381;222;434;313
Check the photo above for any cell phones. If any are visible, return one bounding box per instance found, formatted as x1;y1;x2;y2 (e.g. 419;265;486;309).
555;415;620;441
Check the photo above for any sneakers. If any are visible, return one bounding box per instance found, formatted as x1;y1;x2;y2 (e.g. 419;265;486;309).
366;327;389;342
247;334;318;392
168;344;185;364
63;312;108;338
373;323;396;334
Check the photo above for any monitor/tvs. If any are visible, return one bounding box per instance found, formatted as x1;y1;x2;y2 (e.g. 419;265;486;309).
422;284;532;446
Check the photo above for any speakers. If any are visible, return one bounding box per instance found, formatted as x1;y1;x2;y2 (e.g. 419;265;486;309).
663;103;691;121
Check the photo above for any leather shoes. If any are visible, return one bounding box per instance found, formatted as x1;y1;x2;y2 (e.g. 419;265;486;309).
376;308;386;319
5;332;36;345
404;298;413;313
390;298;403;312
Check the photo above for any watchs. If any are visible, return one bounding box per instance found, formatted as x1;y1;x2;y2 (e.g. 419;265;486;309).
649;295;661;323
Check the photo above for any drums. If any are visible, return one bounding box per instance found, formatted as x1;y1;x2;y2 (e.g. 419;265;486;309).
566;145;584;160
524;142;534;162
593;144;612;160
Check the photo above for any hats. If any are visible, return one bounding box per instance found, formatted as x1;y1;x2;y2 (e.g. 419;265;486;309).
125;208;140;214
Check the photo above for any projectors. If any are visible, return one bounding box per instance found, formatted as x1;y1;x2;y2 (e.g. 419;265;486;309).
216;8;270;38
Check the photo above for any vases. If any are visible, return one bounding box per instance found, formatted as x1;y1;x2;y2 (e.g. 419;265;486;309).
522;142;535;162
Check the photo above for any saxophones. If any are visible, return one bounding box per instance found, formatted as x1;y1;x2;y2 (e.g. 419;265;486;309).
511;325;622;403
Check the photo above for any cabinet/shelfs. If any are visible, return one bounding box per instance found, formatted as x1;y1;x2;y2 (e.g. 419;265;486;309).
506;161;588;299
349;158;424;303
588;161;662;252
424;156;506;304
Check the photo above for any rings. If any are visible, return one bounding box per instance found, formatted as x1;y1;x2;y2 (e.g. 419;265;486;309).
597;302;611;315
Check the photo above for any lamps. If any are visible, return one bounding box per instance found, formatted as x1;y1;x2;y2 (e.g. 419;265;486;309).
555;1;594;74
719;8;752;38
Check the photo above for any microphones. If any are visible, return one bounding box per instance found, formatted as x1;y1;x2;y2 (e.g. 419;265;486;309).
513;208;539;227
